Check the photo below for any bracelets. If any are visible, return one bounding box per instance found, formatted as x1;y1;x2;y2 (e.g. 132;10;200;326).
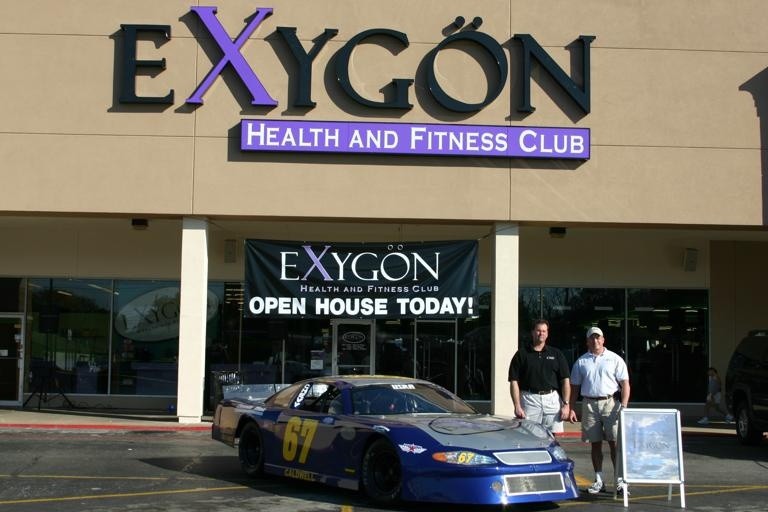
563;400;570;405
621;406;625;408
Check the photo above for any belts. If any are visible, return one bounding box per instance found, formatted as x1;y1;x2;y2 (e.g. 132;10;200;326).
525;388;555;395
585;395;612;400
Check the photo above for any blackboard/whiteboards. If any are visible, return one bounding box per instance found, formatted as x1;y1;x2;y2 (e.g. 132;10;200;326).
620;408;685;484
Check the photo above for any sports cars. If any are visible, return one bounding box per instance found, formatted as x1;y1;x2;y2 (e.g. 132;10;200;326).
211;373;581;510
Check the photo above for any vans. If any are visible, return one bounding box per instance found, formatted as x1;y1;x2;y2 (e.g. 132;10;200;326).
724;329;768;444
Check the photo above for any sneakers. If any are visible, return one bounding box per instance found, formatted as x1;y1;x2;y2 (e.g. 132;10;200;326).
617;480;631;496
588;480;606;494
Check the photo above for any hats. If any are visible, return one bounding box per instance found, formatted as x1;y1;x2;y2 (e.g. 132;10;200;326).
586;327;604;337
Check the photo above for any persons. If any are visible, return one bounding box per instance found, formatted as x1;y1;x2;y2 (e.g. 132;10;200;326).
508;318;571;437
698;367;734;424
565;326;631;498
